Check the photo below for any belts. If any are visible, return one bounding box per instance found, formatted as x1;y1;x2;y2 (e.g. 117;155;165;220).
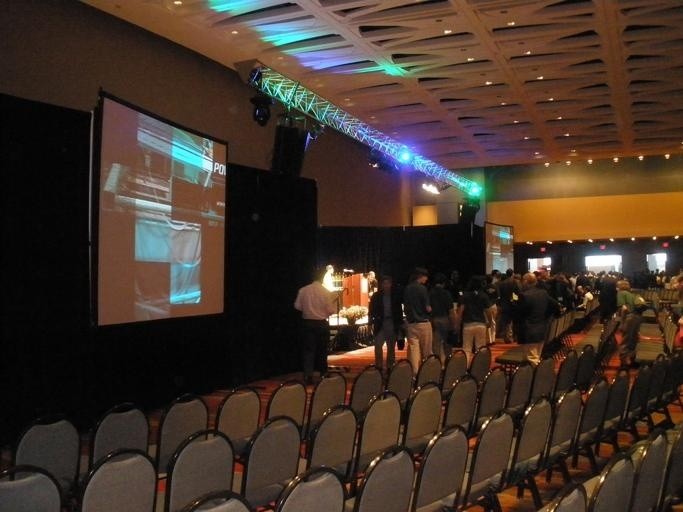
383;315;393;319
407;320;429;324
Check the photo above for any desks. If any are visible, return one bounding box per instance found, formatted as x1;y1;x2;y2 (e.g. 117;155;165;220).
328;312;370;350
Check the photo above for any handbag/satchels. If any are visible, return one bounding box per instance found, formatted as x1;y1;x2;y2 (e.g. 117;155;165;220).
396;335;405;351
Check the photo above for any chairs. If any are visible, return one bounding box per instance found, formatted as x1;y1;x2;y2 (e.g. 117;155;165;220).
351;365;384;410
415;355;443;386
630;364;649;437
167;432;234;511
479;367;508;418
578;345;599;385
243;418;301;505
158;398;208;466
308;405;357;496
411;426;469;511
215;389;262;449
178;493;248;512
507;361;534;414
556;350;578;392
0;467;62;511
387;361;414;399
622;288;682;362
360;392;400;468
444;350;468;383
591;454;634;511
355;445;414;510
507;398;552;510
633;434;667;511
469;346;491;380
669;352;682;413
310;373;346;429
267;381;306;427
606;369;629;453
552;484;588;511
579;379;608;474
442;377;478;431
16;420;81;491
549;388;581;482
461;415;514;510
92;406;150;462
569;320;619;360
82;450;158;511
496;312;572;365
532;359;555;405
649;356;669;432
277;467;346;512
404;383;443;450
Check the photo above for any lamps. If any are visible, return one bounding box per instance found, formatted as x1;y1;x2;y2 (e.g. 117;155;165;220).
423;179;450;195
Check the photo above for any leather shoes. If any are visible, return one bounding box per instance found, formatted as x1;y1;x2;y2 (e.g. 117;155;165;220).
319;371;330;376
491;342;497;345
304;375;315;384
376;366;383;372
387;367;394;371
411;382;416;387
487;343;492;346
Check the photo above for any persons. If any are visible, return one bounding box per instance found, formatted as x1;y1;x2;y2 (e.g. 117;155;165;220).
512;273;566;367
599;270;625;280
364;271;378;299
677;276;683;304
404;267;433;381
367;276;404;371
599;277;617;322
483;275;498;345
630;269;672;291
429;273;455;362
457;276;492;375
497;268;521;344
534;268;599;313
296;265;341;384
616;280;634;311
323;265;339;292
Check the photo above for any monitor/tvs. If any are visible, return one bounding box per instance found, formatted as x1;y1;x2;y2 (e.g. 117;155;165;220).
92;89;228;329
485;222;513;277
